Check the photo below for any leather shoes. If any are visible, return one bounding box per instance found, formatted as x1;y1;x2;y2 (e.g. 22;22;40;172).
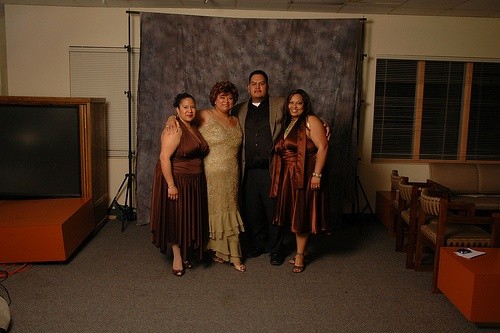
246;248;262;258
271;251;284;265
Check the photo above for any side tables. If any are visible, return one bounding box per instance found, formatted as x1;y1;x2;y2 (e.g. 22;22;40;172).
375;191;390;230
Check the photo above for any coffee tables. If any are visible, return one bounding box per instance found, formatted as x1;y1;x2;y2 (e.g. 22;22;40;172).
437;247;500;326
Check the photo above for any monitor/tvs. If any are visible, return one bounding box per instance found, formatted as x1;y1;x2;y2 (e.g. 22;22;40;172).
0;104;82;198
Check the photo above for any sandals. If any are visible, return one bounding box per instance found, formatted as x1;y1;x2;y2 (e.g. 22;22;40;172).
292;253;305;273
288;249;311;264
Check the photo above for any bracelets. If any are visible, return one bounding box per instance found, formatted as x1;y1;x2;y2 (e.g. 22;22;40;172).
166;125;169;127
168;184;175;189
171;115;176;119
313;172;322;178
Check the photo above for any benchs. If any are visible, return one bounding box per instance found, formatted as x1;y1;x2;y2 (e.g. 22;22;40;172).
428;163;500;211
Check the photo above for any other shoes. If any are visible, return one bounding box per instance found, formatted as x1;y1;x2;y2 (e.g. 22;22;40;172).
172;261;185;276
183;258;193;268
234;264;246;272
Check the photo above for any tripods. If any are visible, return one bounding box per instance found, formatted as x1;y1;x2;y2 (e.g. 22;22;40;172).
108;12;136;231
353;22;375;234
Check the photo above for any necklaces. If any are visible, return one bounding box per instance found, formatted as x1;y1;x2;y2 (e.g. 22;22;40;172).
285;119;296;133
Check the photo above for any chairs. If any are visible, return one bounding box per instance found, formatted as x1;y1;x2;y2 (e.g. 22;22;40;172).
390;169;432;236
395;178;475;269
414;187;496;293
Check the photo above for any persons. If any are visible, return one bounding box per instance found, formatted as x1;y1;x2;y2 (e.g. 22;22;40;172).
166;81;247;272
150;93;210;276
269;89;329;272
231;70;331;265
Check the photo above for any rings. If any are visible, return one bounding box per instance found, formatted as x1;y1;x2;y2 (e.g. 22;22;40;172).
171;195;173;197
315;185;317;187
176;197;178;198
